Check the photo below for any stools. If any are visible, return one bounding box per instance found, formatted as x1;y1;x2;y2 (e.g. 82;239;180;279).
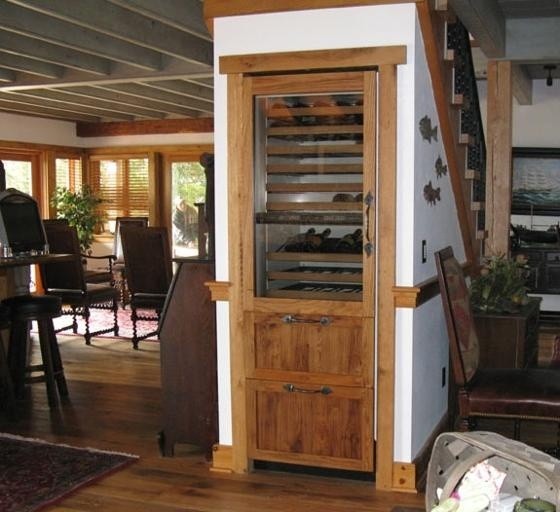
0;295;69;412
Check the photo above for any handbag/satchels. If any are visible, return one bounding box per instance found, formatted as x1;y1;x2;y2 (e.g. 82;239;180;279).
425;431;560;512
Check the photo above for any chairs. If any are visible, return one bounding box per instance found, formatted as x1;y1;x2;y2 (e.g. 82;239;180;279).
434;245;559;441
36;215;171;350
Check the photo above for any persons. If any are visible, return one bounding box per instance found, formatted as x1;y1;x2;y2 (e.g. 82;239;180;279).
171;197;198;248
0;160;34;335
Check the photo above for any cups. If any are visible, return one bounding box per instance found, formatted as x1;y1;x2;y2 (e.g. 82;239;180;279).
0;241;50;259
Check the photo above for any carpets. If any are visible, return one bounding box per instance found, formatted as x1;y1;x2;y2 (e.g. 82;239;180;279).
0;433;139;510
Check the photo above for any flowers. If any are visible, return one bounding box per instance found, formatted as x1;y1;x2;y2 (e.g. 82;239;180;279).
466;249;534;314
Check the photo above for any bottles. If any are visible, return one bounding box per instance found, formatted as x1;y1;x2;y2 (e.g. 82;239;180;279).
266;99;363;141
285;227;364;254
331;193;363;203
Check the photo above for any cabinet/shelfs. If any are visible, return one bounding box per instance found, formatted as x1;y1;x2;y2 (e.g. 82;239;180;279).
475;297;543;368
